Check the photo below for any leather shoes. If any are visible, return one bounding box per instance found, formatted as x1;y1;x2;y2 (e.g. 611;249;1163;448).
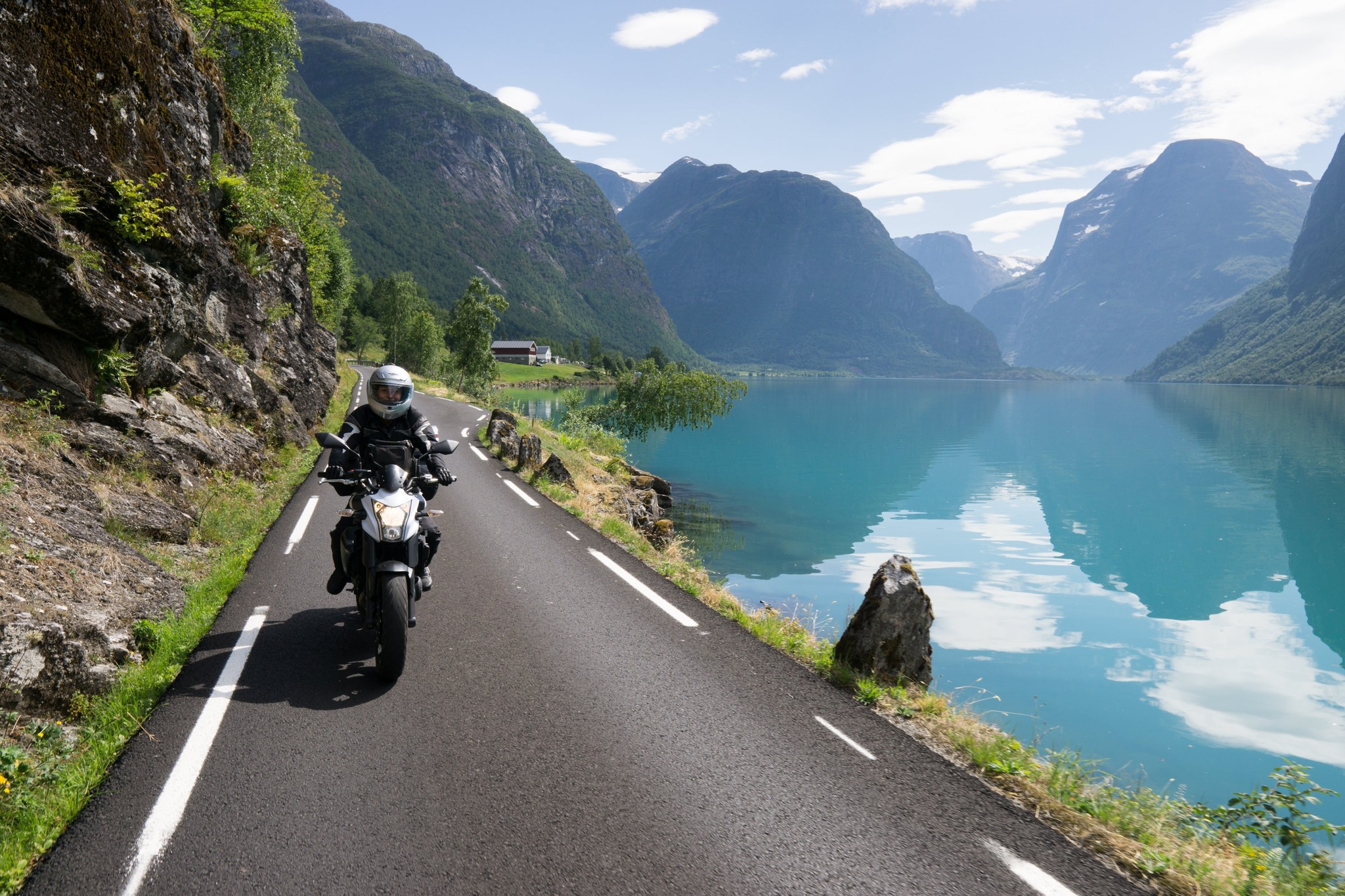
327;566;348;595
420;567;433;592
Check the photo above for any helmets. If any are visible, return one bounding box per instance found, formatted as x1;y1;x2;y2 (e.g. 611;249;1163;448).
366;365;415;420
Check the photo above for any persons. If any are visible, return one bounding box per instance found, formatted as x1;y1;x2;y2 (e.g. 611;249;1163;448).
326;365;452;594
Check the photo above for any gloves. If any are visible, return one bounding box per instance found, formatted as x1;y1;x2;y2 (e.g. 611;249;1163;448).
431;466;453;486
325;464;343;479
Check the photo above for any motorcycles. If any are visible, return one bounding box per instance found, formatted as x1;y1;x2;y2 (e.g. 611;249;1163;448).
314;431;460;677
